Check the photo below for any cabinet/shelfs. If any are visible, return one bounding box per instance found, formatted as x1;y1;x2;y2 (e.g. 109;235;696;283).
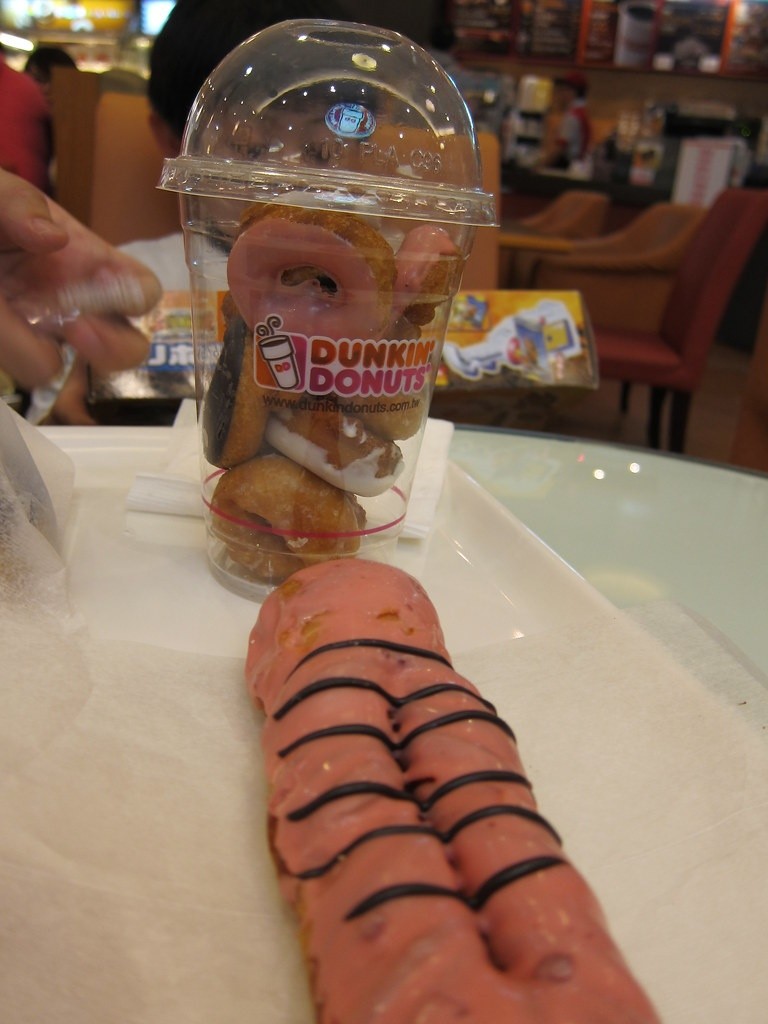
432;49;768;204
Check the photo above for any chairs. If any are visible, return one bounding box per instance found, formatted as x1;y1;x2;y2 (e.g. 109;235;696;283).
498;188;768;454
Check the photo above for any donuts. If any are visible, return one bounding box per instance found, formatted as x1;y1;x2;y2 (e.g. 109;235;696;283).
197;206;459;585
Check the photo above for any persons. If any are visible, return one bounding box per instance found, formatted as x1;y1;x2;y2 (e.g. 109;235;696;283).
0;172;162;426
1;43;80;202
517;71;593;169
148;1;455;141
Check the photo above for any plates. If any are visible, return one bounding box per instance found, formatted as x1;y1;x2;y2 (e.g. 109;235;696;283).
0;436;768;1024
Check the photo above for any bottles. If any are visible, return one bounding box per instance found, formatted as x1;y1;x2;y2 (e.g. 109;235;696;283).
555;83;590;158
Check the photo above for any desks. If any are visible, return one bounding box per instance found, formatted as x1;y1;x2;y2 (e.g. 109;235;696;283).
0;426;768;1024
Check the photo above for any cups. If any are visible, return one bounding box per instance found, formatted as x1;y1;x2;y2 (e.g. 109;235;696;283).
164;19;496;596
